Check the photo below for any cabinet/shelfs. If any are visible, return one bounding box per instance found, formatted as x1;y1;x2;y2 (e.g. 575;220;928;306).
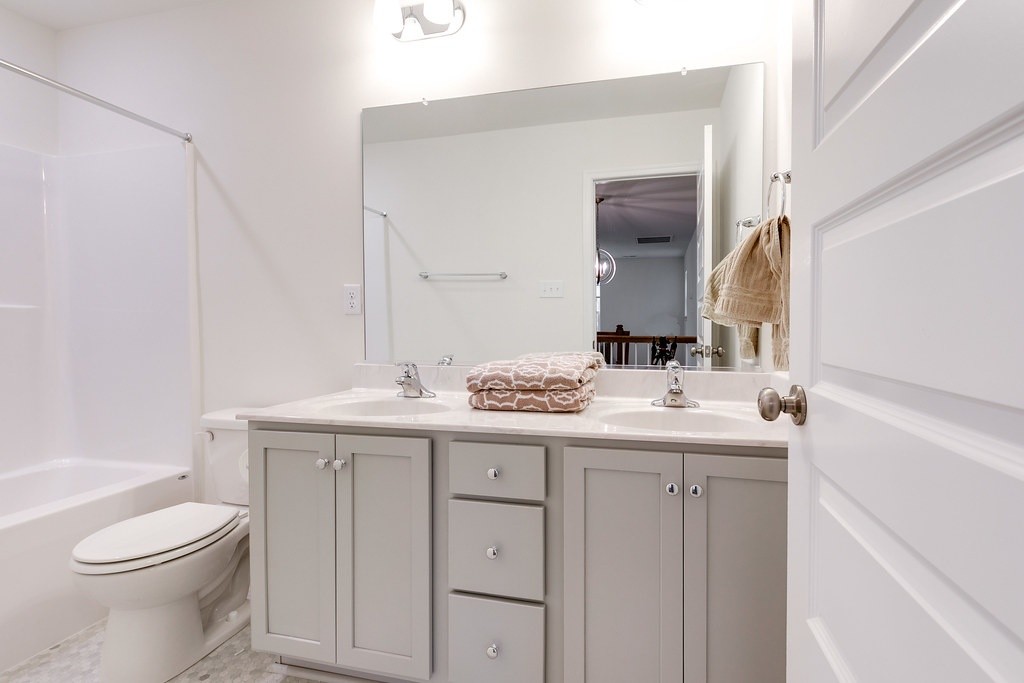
561;443;788;682
249;427;433;680
445;428;551;682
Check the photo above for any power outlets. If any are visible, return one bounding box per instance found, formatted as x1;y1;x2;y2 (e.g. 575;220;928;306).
541;280;564;298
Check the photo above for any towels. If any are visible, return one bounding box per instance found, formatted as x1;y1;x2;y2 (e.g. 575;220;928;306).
715;217;789;370
468;380;596;411
465;350;606;392
701;246;758;361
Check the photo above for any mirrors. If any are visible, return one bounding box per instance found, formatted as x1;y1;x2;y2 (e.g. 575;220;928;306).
361;61;763;371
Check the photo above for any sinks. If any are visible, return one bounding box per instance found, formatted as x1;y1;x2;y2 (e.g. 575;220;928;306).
307;397;457;417
600;409;762;433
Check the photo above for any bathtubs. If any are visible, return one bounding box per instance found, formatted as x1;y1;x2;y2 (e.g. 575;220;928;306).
2;454;196;675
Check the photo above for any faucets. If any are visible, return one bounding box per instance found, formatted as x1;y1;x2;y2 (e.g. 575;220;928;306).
650;359;701;407
394;360;433;399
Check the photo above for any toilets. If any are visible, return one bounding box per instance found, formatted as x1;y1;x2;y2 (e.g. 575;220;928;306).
70;409;250;668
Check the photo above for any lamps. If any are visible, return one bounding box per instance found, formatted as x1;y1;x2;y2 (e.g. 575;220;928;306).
594;196;617;285
373;0;465;41
